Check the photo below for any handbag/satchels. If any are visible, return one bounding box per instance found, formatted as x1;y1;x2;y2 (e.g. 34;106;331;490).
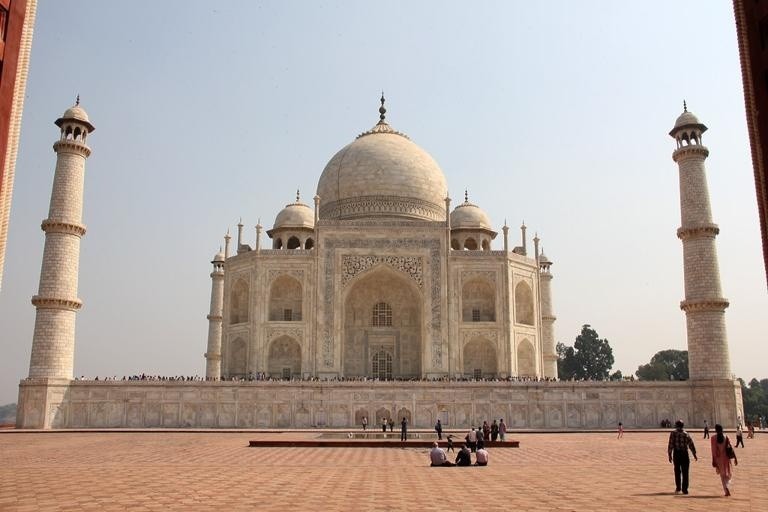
727;447;734;458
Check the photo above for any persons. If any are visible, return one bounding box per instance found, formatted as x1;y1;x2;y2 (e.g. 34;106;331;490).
747;421;756;438
465;418;508;453
400;416;408;441
473;445;489;466
668;420;698;494
435;418;442;439
430;441;456;466
736;416;743;430
711;424;738;496
734;427;745;448
661;418;672;428
617;422;624;439
702;420;710;440
381;417;394;432
80;373;557;382
446;436;454;452
455;444;474;466
362;416;366;430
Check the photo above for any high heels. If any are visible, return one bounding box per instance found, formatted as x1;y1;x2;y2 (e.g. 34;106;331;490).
725;493;730;496
